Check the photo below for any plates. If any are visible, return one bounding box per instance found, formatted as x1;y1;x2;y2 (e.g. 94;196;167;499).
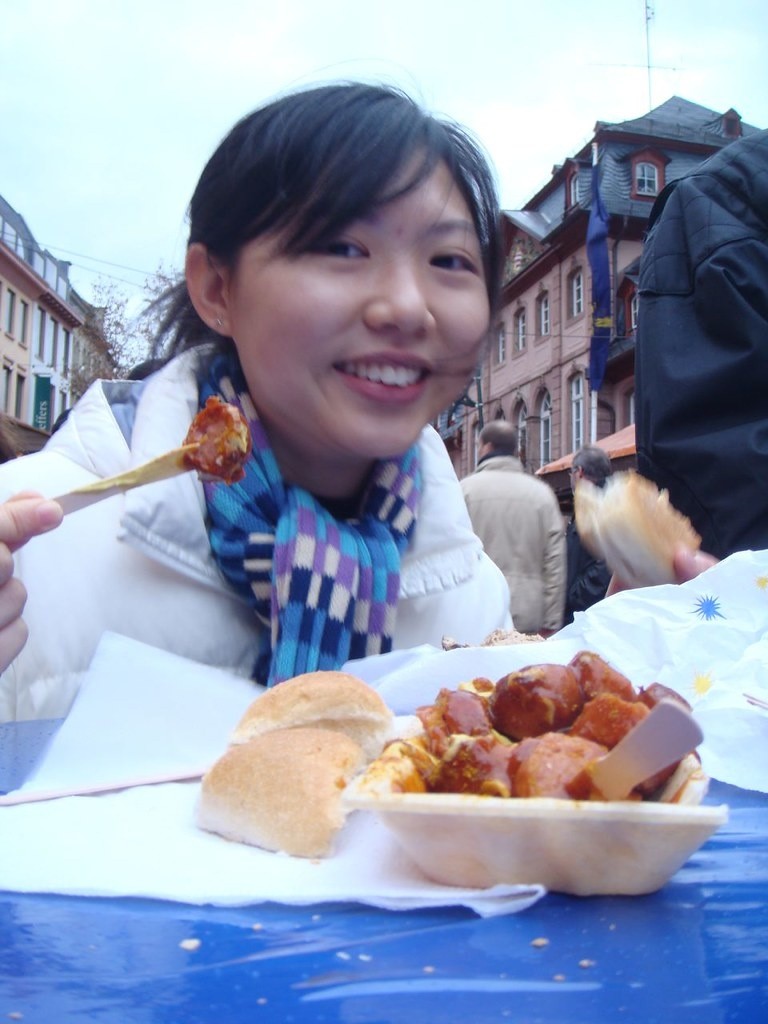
342;791;729;897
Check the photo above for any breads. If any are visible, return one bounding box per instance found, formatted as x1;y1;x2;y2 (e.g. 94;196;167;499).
196;672;393;861
571;471;703;586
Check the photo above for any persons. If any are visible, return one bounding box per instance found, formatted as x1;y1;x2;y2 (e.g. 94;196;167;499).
459;421;615;640
0;80;722;722
634;129;768;560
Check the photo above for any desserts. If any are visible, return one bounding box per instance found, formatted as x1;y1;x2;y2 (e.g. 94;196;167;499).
369;650;711;813
185;400;254;480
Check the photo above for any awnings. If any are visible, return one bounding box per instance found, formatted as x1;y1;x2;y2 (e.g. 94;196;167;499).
535;424;636;476
